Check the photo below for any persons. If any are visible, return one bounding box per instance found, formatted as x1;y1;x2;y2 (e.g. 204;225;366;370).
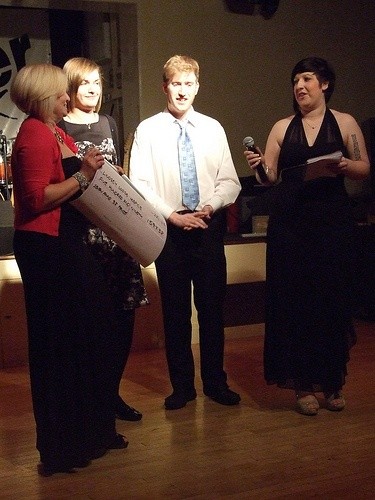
244;58;371;416
128;55;241;409
56;57;151;449
10;64;125;471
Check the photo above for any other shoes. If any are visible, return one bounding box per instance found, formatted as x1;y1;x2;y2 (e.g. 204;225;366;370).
294;392;320;415
108;433;129;449
203;389;242;406
115;394;142;421
322;387;345;410
164;389;197;410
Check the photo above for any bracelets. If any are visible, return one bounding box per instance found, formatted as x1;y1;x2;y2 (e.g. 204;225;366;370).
266;166;269;174
73;172;88;189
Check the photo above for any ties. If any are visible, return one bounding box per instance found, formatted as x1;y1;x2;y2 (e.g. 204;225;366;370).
176;119;201;212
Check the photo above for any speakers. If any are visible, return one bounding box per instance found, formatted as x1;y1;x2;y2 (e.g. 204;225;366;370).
0;201;16;256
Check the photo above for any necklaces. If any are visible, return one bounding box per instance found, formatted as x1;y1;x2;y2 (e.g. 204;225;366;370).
302;112;324;129
52;129;64;144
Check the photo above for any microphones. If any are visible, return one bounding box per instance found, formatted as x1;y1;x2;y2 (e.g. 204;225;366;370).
243;136;269;183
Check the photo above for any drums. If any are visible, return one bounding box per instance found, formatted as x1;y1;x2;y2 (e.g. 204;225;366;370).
0;161;12;180
